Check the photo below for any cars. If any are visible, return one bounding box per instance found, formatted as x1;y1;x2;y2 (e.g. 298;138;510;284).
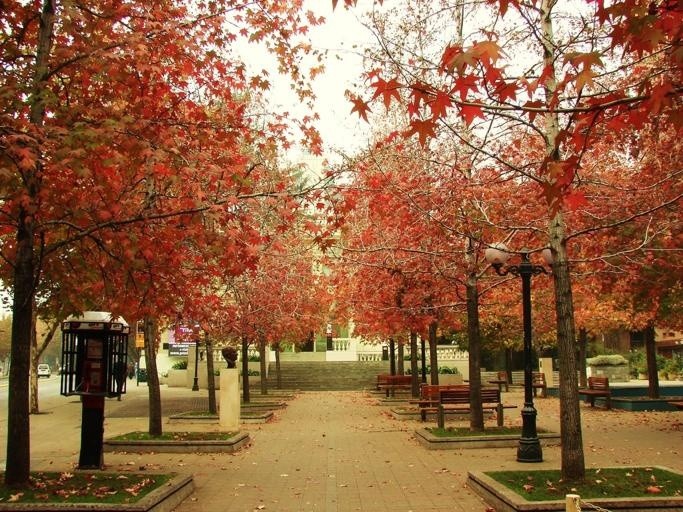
57;363;75;375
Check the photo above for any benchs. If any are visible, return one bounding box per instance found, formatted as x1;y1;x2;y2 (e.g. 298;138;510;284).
520;372;547;398
577;377;612;410
375;371;510;426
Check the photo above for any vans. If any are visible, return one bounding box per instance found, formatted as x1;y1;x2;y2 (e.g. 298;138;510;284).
37;363;50;378
